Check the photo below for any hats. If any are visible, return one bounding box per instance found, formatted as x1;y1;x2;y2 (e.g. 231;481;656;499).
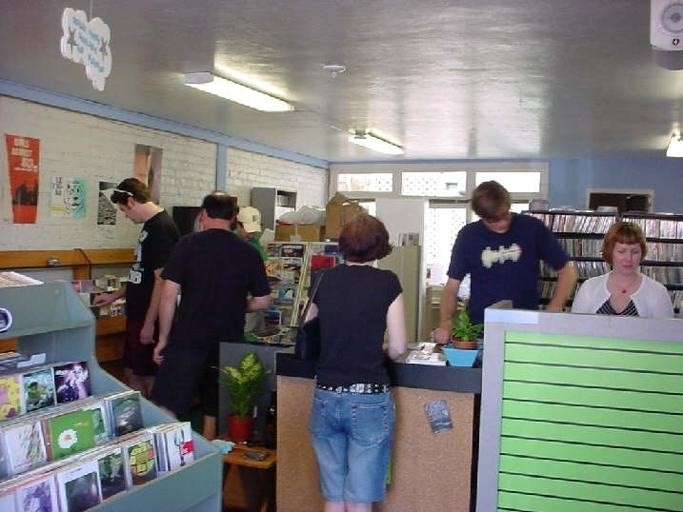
236;206;262;235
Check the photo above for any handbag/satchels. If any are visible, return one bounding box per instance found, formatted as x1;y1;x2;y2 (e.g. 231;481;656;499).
294;267;322;362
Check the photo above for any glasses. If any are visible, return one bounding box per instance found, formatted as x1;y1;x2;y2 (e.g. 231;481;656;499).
110;188;133;197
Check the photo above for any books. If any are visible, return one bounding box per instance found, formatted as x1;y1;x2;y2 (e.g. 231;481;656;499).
523;208;682;319
0;359;196;511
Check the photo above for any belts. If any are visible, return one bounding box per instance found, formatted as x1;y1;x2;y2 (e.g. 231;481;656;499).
313;378;393;394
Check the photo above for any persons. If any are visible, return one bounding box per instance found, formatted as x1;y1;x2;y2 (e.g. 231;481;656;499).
293;215;407;512
91;177;182;397
428;180;578;346
149;191;271;439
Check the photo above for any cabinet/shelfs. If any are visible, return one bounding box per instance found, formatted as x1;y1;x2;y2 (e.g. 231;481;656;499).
250;187;299;241
0;278;224;512
254;242;346;330
520;209;683;313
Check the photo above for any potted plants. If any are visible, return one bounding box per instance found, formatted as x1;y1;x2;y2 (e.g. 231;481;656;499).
208;352;277;442
444;310;484;350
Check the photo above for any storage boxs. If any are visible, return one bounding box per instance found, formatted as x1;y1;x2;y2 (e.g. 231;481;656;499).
275;191;369;241
